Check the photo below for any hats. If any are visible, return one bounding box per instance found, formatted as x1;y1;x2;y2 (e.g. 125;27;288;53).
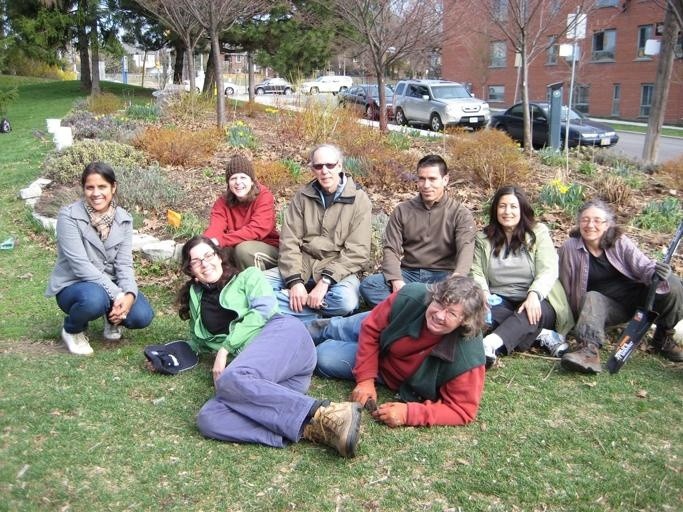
226;154;255;181
144;340;199;375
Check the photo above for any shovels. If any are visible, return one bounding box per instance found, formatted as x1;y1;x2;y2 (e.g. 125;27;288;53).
606;219;683;376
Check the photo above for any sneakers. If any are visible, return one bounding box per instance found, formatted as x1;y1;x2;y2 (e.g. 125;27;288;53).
61;327;94;355
104;314;124;339
538;330;569;357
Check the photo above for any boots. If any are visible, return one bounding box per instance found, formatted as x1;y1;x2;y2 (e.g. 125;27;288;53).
653;329;683;362
561;343;602;374
302;401;363;459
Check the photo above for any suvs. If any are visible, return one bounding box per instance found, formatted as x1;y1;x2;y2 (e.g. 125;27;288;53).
390;79;490;132
301;75;352;96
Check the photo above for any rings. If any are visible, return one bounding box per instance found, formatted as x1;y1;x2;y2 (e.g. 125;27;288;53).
119;313;126;320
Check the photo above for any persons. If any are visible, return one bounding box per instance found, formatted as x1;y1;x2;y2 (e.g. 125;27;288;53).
201;154;280;272
171;235;363;458
313;271;493;430
358;155;477;311
556;199;683;376
465;184;577;372
273;139;372;321
43;162;155;355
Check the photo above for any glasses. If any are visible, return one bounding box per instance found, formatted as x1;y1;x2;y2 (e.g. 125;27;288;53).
189;250;217;269
579;218;607;226
313;162;338;170
435;301;464;323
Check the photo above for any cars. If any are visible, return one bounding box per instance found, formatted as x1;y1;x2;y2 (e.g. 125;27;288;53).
247;78;296;96
489;99;618;150
337;83;394;121
183;76;239;95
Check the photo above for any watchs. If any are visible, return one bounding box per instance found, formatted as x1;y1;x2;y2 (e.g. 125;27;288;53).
322;273;332;284
